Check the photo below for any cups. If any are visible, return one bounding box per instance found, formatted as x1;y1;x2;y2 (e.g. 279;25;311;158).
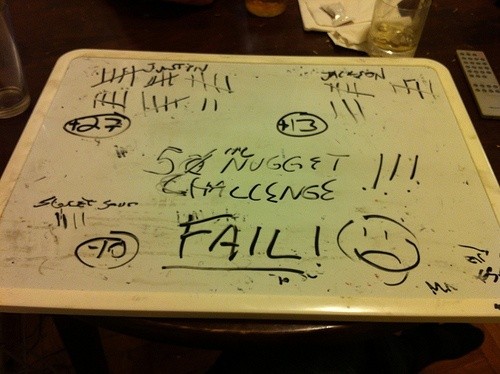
368;0;431;58
0;17;30;119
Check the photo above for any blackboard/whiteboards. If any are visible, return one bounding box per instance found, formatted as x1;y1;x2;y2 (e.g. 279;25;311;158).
0;48;499;325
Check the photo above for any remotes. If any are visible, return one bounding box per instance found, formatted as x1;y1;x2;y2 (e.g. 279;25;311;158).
457;50;500;118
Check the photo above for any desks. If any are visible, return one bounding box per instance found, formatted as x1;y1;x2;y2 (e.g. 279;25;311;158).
0;1;500;374
